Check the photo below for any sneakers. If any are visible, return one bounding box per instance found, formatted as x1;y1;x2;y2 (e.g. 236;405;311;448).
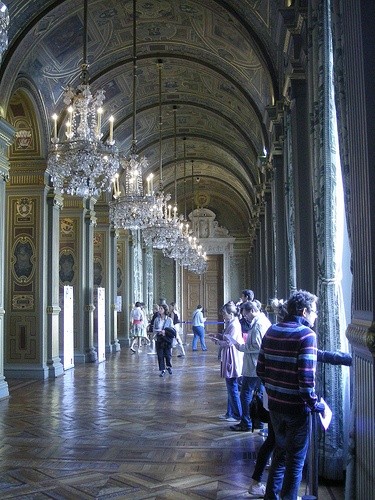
248;483;266;497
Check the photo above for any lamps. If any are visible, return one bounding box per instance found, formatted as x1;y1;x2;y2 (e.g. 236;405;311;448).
0;0;208;276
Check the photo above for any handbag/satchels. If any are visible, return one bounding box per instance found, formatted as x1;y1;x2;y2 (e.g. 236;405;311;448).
249;394;270;423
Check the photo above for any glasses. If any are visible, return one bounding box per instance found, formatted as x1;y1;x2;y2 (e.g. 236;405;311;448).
299;307;319;315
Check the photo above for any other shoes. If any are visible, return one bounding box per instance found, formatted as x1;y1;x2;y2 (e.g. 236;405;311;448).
166;367;172;374
160;371;165;377
138;350;142;353
177;354;184;357
130;348;136;352
229;422;252;432
225;416;240;421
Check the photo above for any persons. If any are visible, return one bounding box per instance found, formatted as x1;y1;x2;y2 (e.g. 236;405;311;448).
130;301;150;352
170;302;186;357
248;289;352;500
153;303;174;377
208;289;272;432
148;303;161;354
191;304;207;352
